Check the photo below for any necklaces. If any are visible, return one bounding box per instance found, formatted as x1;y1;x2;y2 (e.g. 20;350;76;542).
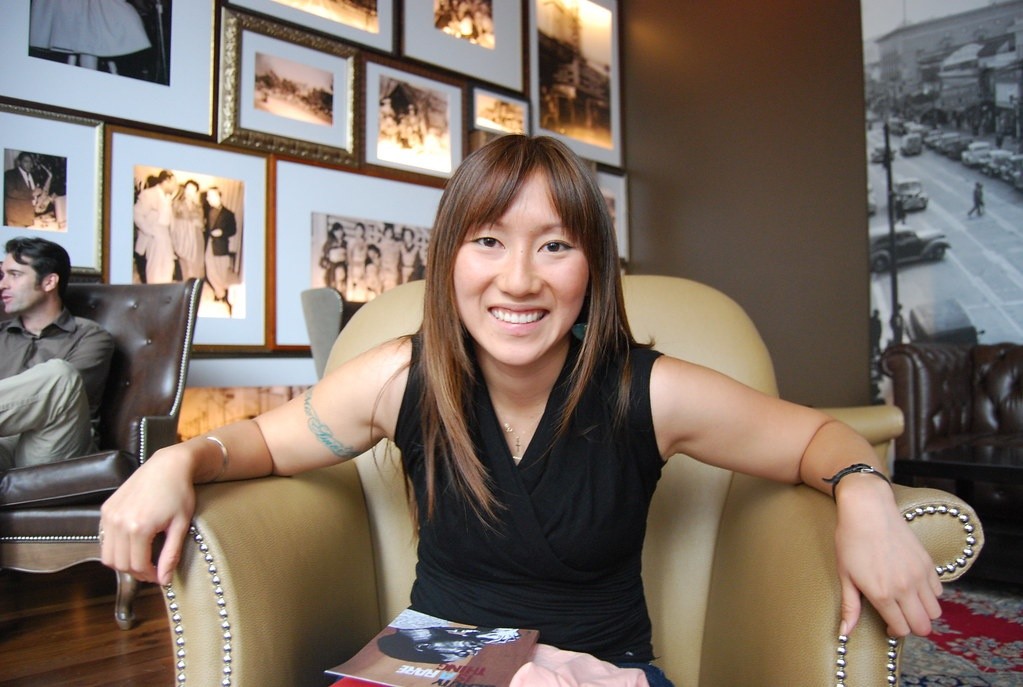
499;412;543;452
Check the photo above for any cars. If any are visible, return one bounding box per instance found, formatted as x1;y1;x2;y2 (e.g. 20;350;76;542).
870;224;951;274
889;114;1022;190
895;178;932;213
903;296;992;346
872;146;897;166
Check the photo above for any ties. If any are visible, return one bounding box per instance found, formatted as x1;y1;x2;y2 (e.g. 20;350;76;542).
27;173;31;189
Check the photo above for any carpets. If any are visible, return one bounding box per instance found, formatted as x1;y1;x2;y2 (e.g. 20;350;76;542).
897;574;1023;687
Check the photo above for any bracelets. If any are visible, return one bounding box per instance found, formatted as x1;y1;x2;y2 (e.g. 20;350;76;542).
823;464;891;504
206;435;229;482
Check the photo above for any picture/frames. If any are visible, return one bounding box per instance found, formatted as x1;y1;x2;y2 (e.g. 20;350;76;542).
0;0;630;358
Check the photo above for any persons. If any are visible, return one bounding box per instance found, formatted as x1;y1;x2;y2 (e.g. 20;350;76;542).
320;221;423;302
968;182;985;218
4;152;41;228
995;131;1004;149
380;98;420;148
871;308;883;354
896;200;905;224
101;133;944;687
435;0;492;42
890;303;904;343
0;235;116;482
132;170;238;302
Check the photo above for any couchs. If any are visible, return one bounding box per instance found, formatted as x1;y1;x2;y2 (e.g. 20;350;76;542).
879;339;1022;591
0;262;204;629
159;275;984;684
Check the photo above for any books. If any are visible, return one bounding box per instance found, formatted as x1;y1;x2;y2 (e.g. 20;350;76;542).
324;609;541;687
